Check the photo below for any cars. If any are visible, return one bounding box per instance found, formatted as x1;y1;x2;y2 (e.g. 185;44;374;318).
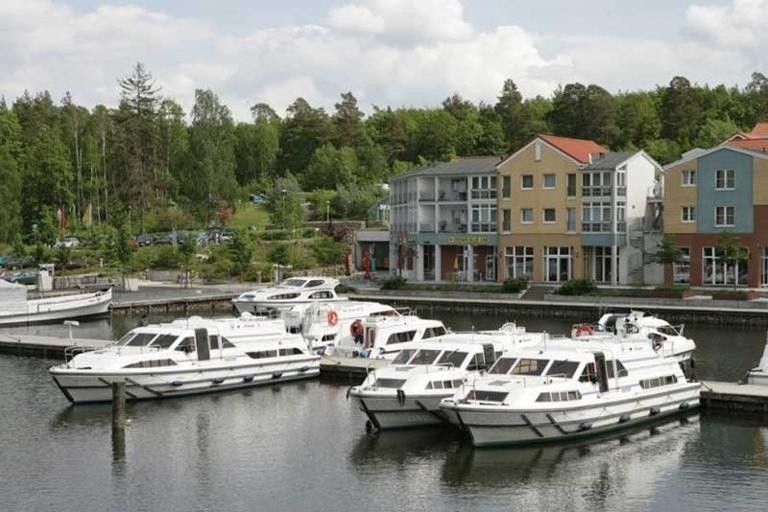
136;229;236;246
60;237;80;247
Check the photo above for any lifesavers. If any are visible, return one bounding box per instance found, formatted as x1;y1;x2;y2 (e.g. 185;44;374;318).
575;325;593;342
328;311;338;325
351;321;362;337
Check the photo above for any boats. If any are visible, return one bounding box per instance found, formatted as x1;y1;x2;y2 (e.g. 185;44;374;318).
0;279;113;323
50;315;321;406
236;276;451;378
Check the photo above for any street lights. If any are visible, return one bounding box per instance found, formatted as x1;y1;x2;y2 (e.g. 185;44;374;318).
281;189;287;232
